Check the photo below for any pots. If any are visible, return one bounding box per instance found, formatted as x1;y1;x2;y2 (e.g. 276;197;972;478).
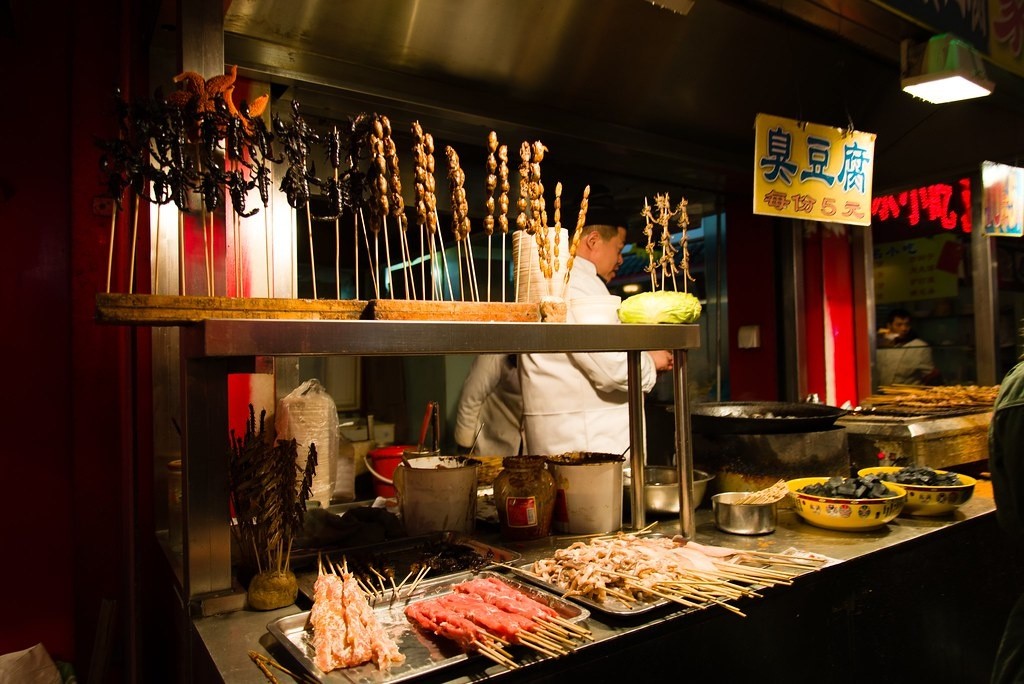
665;400;850;437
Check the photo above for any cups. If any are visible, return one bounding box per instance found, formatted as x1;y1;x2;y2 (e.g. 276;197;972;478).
168;459;183;555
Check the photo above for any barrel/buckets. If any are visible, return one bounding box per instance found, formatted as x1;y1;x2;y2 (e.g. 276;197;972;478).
394;456;482;539
366;445;441;498
543;451;629;534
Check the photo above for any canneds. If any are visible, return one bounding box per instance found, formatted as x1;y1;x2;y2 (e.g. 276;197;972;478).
494;454;556;542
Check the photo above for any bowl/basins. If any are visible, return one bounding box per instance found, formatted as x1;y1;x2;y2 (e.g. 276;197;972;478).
286;397;331;511
785;475;907;533
856;466;978;518
511;228;572;303
621;465;716;517
710;491;778;535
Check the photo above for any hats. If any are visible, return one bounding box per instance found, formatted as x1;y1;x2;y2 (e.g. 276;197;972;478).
567;185;627;229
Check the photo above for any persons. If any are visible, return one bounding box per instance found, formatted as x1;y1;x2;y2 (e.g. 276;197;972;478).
454;350;529;463
517;223;676;487
875;309;937;390
987;317;1024;684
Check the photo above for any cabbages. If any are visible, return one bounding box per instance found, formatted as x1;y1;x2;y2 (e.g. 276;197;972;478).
616;290;702;324
